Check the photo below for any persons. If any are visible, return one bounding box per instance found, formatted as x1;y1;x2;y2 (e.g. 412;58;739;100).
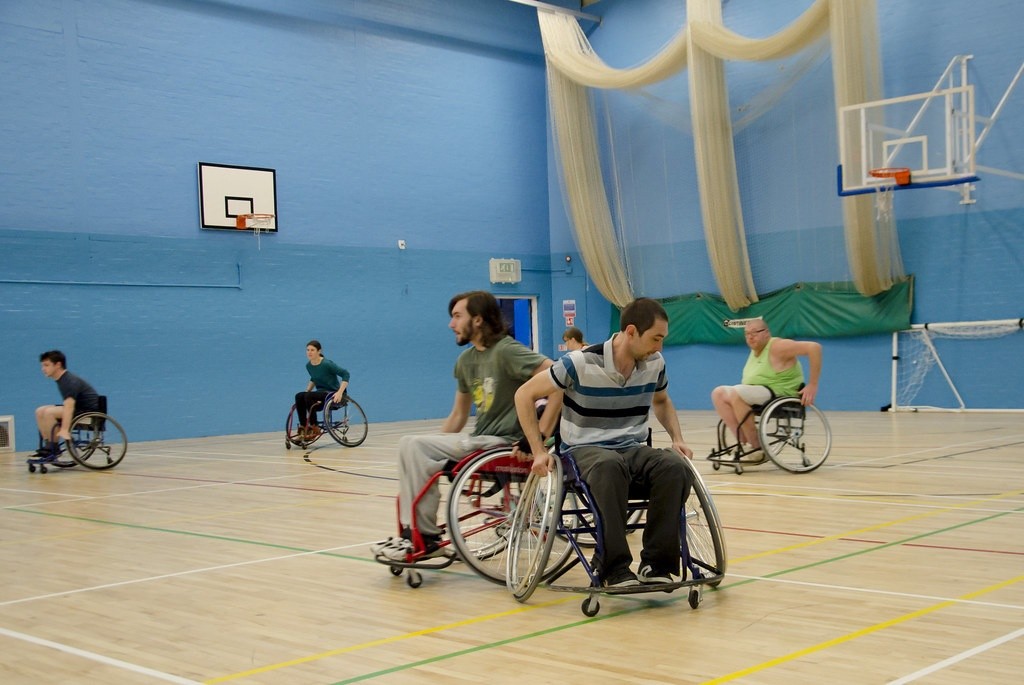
27;350;101;459
711;318;823;461
369;290;563;561
561;325;590;351
290;340;350;442
514;297;693;593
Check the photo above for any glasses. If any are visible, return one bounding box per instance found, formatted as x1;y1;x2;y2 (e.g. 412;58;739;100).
744;329;764;337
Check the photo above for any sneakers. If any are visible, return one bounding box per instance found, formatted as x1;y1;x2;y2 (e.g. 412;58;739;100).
602;566;640;587
636;562;673;583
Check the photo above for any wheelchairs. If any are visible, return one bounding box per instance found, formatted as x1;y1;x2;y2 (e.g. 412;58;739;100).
26;395;128;474
285;388;368;450
375;404;727;617
706;383;832;475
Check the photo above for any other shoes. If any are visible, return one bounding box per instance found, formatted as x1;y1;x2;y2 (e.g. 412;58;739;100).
382;539;445;561
713;442;744;461
287;427;305;442
305;426;321;440
28;447;62;461
740;443;763;462
371;536;402;555
